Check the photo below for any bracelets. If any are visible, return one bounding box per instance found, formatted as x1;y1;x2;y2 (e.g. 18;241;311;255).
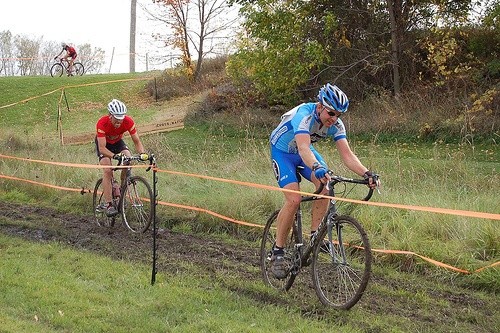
113;155;115;159
140;153;145;155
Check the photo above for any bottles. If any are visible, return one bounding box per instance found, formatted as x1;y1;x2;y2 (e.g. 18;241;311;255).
113;180;120;197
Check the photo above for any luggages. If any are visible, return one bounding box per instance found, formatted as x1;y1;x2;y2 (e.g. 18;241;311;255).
124;198;129;209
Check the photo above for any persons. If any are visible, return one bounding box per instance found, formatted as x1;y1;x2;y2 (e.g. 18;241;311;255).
268;83;383;279
95;99;149;217
54;42;77;76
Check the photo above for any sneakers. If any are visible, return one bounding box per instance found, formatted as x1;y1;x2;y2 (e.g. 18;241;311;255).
271;242;286;278
310;233;334;253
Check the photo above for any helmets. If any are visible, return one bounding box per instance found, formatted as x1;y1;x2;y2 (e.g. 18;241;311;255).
61;42;66;48
316;82;349;113
107;99;127;119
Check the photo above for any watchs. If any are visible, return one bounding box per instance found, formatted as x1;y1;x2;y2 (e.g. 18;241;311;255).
311;162;320;170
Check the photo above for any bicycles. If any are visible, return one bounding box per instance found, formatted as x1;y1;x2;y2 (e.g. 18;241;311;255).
51;56;84;78
92;152;153;235
260;169;382;311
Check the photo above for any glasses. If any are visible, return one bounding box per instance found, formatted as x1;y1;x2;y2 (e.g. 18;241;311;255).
321;104;343;118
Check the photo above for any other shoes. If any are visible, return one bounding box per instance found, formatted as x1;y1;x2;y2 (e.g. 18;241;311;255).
106;207;118;217
